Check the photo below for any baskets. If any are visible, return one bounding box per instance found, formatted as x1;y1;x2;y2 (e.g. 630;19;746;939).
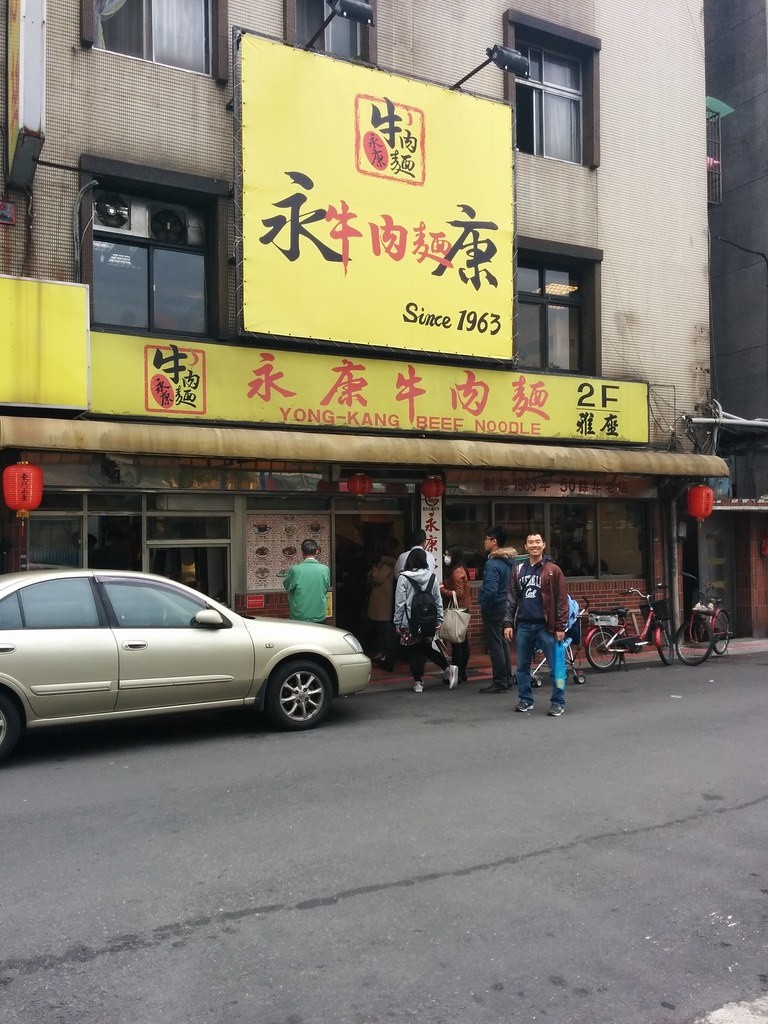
639;599;671;623
692;589;715;616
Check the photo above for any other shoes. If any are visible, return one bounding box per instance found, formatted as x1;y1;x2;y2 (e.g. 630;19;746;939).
443;666;467;684
413;681;424;692
480;683;512;692
449;665;458;690
369;654;395;671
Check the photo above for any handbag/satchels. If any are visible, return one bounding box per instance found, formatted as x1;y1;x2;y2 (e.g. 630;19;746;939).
438;590;471;644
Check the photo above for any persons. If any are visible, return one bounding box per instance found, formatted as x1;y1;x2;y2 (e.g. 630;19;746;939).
367;537;400;670
503;531;569;716
478;525;518;693
568;547;592;576
284;540;331;624
601;559;612;575
439;545;471;684
394;529;435;579
393;549;458;692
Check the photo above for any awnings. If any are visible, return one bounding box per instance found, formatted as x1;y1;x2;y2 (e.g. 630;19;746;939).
0;414;730;477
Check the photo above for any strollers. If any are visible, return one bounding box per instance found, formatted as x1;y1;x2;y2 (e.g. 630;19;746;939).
511;593;590;688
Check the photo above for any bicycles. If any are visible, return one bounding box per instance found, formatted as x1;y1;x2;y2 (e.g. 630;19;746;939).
584;582;675;673
674;572;733;666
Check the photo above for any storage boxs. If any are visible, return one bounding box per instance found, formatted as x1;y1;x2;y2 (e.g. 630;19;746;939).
589;610;619;625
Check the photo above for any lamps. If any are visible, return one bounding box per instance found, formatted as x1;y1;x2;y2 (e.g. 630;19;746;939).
304;0;377;51
449;44;531;89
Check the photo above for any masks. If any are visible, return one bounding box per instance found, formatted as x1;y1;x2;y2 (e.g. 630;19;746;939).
444;556;451;566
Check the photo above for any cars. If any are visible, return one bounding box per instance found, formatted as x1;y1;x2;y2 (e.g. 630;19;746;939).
0;565;373;768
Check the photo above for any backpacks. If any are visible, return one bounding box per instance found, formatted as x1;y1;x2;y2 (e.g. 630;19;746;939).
401;573;437;637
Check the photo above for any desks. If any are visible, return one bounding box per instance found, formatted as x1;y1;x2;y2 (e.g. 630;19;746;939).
576;609;648;651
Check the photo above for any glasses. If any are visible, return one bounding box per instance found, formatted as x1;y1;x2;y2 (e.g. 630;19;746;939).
484;537;493;541
443;554;450;557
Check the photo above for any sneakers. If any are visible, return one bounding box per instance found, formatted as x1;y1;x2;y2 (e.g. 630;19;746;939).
515;701;533;712
547;704;564;716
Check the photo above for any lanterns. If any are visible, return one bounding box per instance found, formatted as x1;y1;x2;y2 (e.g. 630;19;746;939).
3;461;43;536
317;479;340;492
386;483;409;493
687;484;714;522
421;475;445;501
348;472;372;497
